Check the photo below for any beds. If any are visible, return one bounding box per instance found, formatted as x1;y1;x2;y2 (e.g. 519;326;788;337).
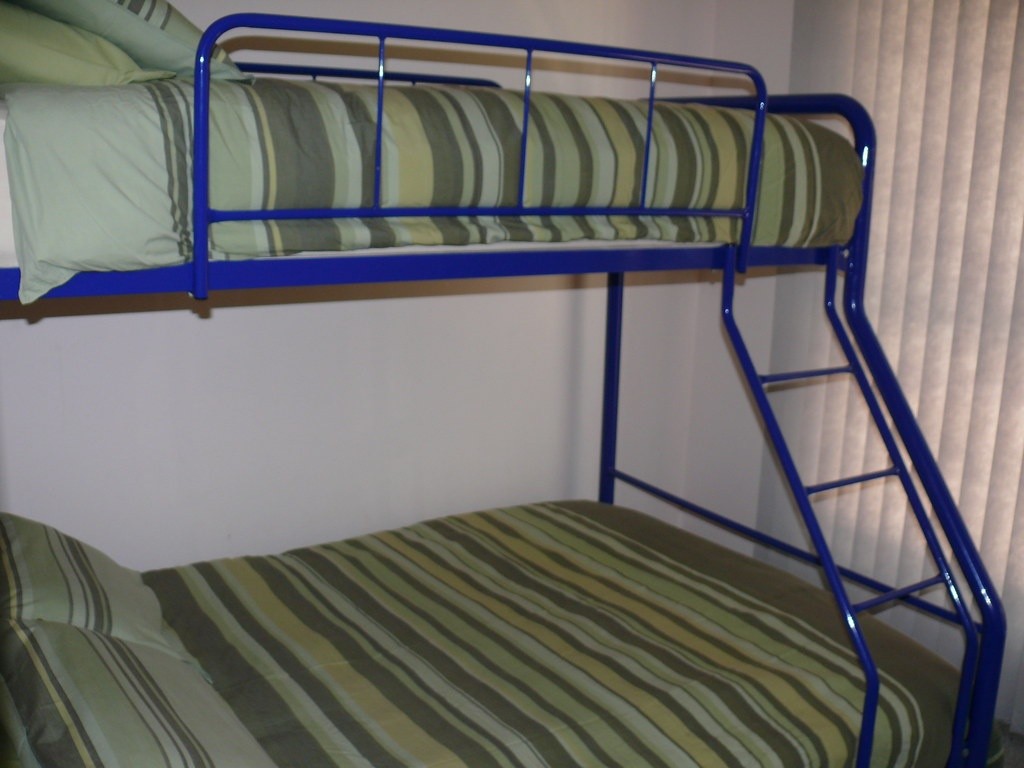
0;12;1005;768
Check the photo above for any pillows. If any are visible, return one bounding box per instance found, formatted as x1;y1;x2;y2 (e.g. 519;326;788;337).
0;1;177;87
11;0;252;80
0;512;217;688
0;617;280;768
